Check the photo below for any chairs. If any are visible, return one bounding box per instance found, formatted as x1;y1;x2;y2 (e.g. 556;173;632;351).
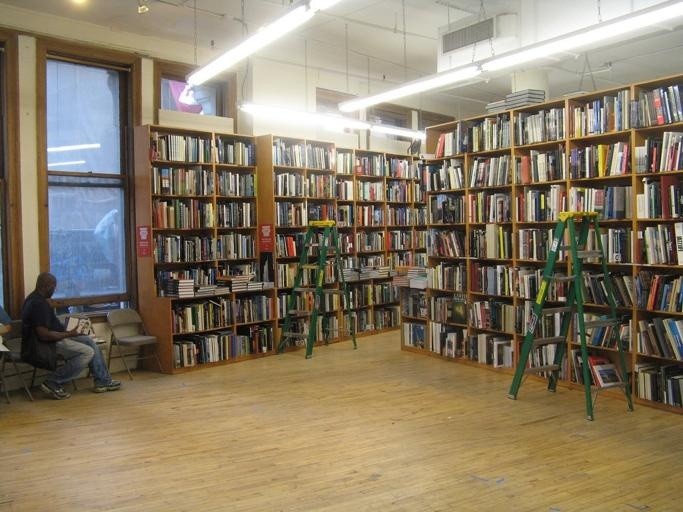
0;308;165;405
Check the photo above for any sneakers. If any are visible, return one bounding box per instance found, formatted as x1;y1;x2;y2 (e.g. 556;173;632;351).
40;381;72;400
94;380;120;393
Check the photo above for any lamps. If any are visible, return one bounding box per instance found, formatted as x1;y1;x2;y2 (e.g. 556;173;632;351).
184;0;341;87
339;0;683;114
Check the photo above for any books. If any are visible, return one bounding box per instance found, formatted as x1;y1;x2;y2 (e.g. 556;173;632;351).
427;88;681;407
149;131;430;367
91;337;107;344
65;317;91;336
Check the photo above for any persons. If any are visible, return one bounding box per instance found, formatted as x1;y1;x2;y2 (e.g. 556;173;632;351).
0;304;12;334
21;273;122;400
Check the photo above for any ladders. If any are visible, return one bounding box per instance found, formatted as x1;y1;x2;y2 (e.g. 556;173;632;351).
278;221;357;359
508;212;637;420
